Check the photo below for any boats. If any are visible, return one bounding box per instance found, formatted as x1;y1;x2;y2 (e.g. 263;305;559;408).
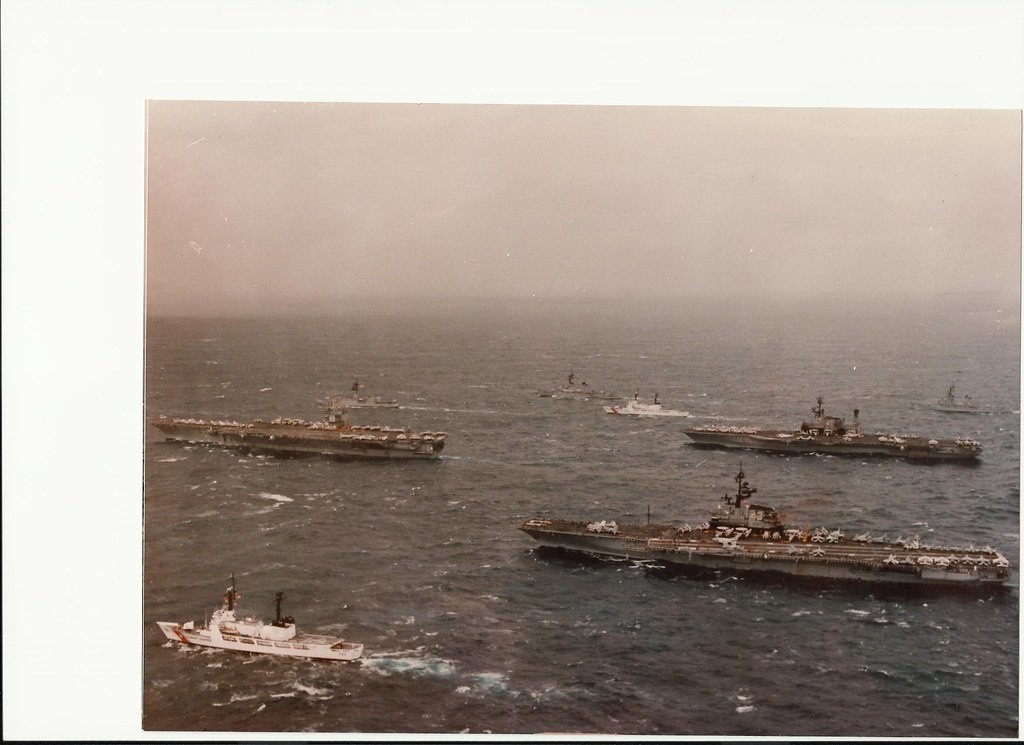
149;396;450;462
680;390;983;459
927;387;1013;415
325;379;400;410
154;573;364;660
517;457;1010;593
552;383;625;400
601;400;690;418
